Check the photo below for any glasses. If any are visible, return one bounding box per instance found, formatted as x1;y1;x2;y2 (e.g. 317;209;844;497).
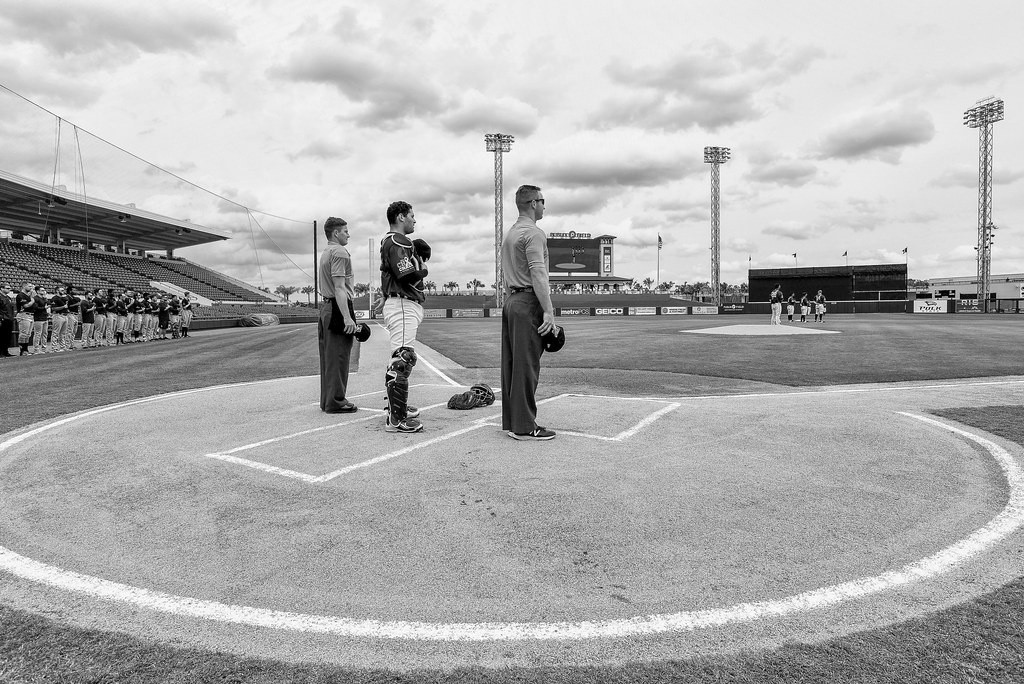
526;198;545;206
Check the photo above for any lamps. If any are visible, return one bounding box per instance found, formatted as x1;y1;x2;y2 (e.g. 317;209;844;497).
120;216;126;222
178;229;183;235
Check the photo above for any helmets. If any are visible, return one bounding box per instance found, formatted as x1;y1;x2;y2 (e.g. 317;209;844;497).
470;383;495;407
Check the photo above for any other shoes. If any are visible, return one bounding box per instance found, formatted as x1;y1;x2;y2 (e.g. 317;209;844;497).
332;404;358;413
347;403;355;406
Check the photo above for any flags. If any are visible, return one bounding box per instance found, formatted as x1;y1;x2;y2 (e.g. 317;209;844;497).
842;251;847;257
902;248;907;254
658;236;663;250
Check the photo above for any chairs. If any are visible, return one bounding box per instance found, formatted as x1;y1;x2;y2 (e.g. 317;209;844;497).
0;240;321;322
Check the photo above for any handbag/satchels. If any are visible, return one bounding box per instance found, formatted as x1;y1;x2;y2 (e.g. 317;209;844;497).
328;297;356;334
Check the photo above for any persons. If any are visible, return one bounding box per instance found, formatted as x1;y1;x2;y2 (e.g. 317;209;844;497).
373;201;428;432
787;292;796;322
800;292;811;323
317;217;358;414
769;283;783;325
814;290;826;323
0;284;193;358
500;185;557;441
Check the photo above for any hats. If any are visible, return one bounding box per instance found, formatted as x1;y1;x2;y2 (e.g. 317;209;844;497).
351;323;371;342
542;325;565;353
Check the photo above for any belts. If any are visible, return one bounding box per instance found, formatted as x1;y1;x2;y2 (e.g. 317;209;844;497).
511;287;534;293
386;292;421;305
325;298;332;303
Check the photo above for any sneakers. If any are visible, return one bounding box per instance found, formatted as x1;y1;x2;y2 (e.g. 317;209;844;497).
384;414;423;433
507;429;514;437
407;406;420;418
513;426;556;440
